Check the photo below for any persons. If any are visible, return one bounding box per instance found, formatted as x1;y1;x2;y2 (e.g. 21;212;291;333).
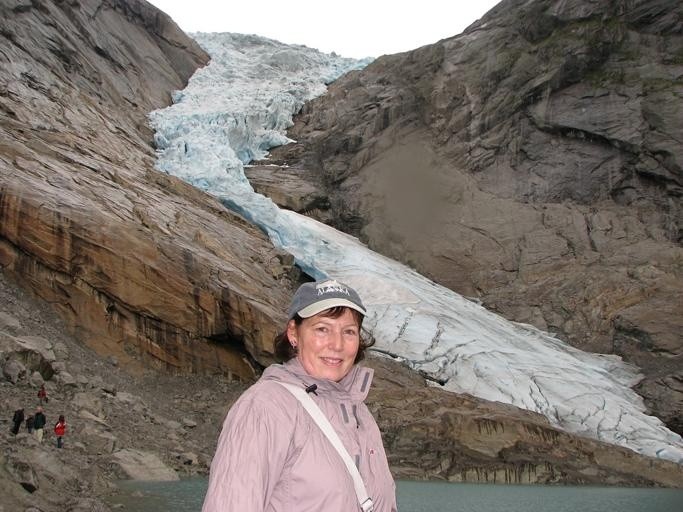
53;414;65;448
26;411;35;436
199;279;398;512
33;406;47;442
36;382;48;407
9;406;25;436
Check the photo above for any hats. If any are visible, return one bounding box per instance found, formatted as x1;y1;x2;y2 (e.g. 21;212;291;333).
288;281;369;323
